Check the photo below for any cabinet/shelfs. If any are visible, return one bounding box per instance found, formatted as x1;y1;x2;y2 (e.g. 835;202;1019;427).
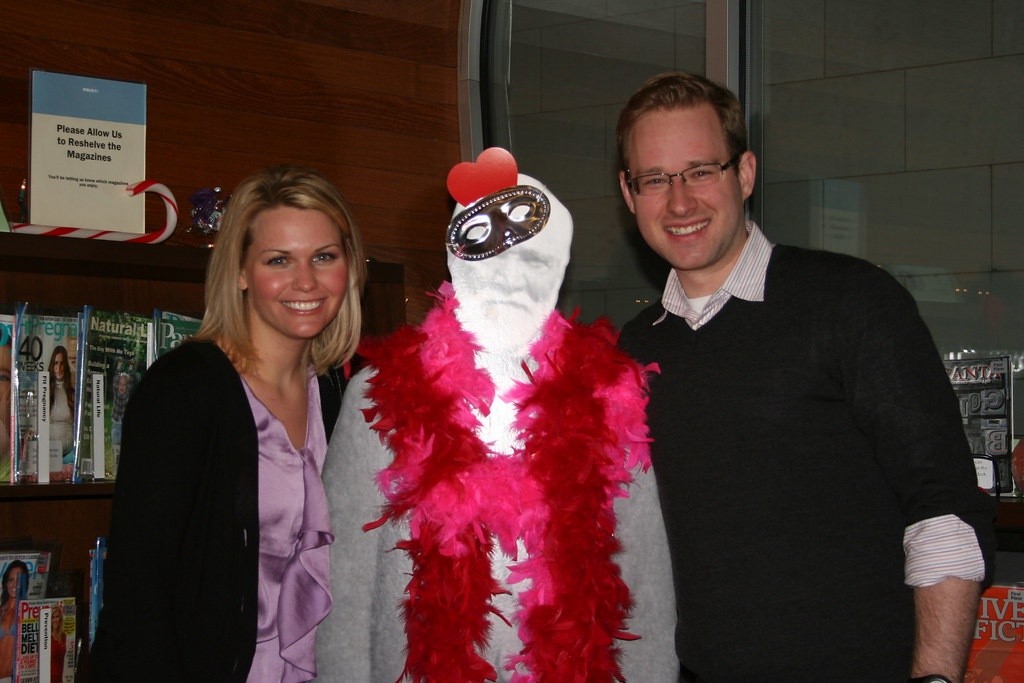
0;232;403;683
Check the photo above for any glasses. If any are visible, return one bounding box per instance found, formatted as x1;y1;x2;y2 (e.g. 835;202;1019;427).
626;149;744;196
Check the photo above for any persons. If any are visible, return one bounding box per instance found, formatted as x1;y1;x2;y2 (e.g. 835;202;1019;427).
82;162;370;683
316;146;680;683
0;336;11;457
49;346;74;454
112;362;130;475
51;607;67;683
0;561;28;678
618;72;992;683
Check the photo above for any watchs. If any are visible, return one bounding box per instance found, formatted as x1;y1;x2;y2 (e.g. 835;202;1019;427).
911;674;952;683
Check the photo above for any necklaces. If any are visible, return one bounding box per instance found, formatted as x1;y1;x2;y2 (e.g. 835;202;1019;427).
359;280;661;683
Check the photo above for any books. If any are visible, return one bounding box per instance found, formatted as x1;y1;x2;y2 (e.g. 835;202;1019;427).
0;304;202;485
0;537;106;683
